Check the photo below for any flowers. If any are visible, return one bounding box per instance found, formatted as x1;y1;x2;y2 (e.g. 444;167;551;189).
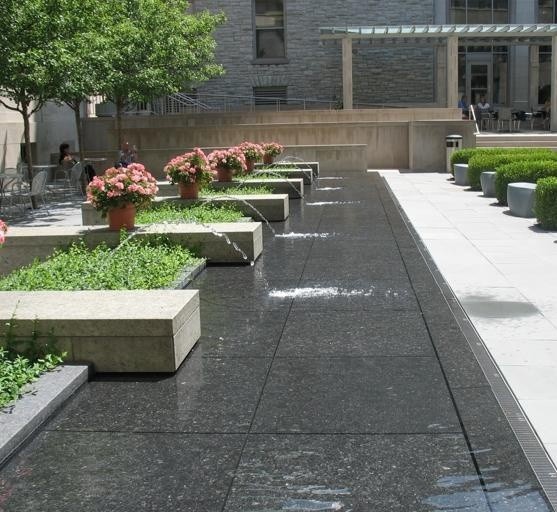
207;147;247;171
164;147;211;183
260;142;283;155
0;220;8;248
240;142;265;163
85;162;159;219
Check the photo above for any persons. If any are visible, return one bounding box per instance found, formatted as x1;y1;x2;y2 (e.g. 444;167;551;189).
477;95;490;113
543;97;551;131
58;143;96;196
119;141;135;167
459;95;487;131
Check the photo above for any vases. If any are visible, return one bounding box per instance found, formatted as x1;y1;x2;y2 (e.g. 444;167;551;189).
245;160;254;171
264;155;273;164
177;183;199;198
217;171;233;181
106;201;136;231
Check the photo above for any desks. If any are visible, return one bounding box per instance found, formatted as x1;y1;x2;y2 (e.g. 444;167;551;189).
478;105;550;133
84;157;107;168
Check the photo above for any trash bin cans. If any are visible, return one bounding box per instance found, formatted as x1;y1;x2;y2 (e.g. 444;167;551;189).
445;134;463;173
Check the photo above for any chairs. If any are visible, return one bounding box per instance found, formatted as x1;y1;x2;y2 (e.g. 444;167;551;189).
0;155;83;217
